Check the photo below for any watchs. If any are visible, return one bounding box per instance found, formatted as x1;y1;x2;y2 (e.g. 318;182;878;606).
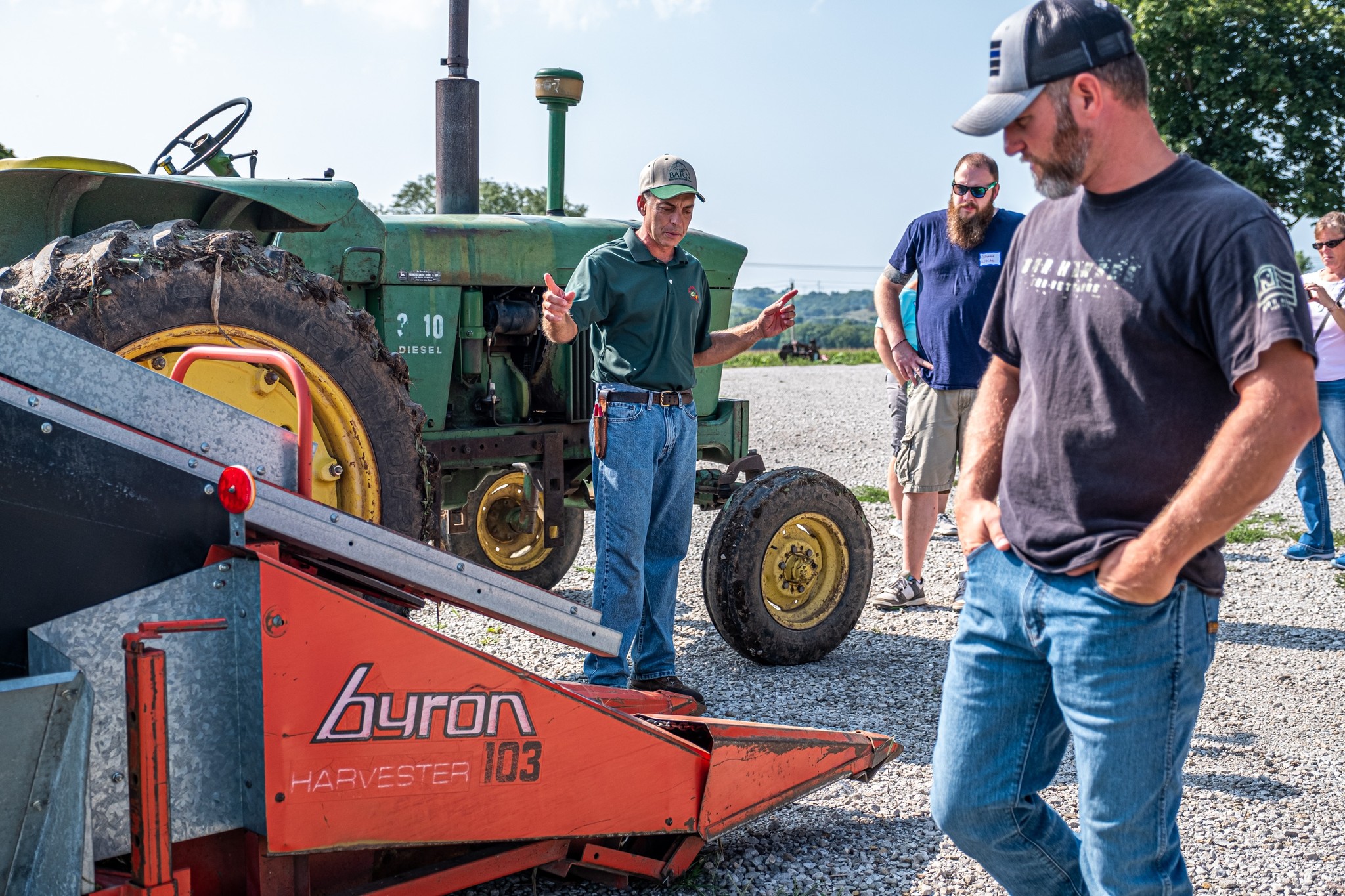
1327;301;1342;313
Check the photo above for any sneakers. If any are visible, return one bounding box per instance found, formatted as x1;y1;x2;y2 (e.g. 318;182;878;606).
1331;553;1345;569
890;518;905;539
629;676;707;707
951;570;970;610
1282;544;1335;561
933;513;958;535
873;570;927;608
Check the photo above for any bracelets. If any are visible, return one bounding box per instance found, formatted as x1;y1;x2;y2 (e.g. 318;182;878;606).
891;338;908;352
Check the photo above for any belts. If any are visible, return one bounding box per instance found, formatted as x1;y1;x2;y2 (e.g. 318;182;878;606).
598;388;694;407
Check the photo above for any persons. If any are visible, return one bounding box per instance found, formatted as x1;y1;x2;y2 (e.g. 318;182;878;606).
872;152;1027;609
542;152;799;701
930;0;1322;896
873;279;958;540
1281;210;1345;570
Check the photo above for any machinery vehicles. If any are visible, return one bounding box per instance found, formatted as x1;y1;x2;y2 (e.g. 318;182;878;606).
0;301;904;896
0;0;875;668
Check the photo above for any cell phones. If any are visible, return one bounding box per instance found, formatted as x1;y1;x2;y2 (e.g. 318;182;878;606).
1304;290;1312;300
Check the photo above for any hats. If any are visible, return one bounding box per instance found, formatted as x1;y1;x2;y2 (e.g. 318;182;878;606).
952;0;1138;136
639;152;706;203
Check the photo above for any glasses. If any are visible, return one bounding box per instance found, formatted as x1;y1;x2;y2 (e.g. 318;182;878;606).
1312;237;1345;250
950;179;998;198
